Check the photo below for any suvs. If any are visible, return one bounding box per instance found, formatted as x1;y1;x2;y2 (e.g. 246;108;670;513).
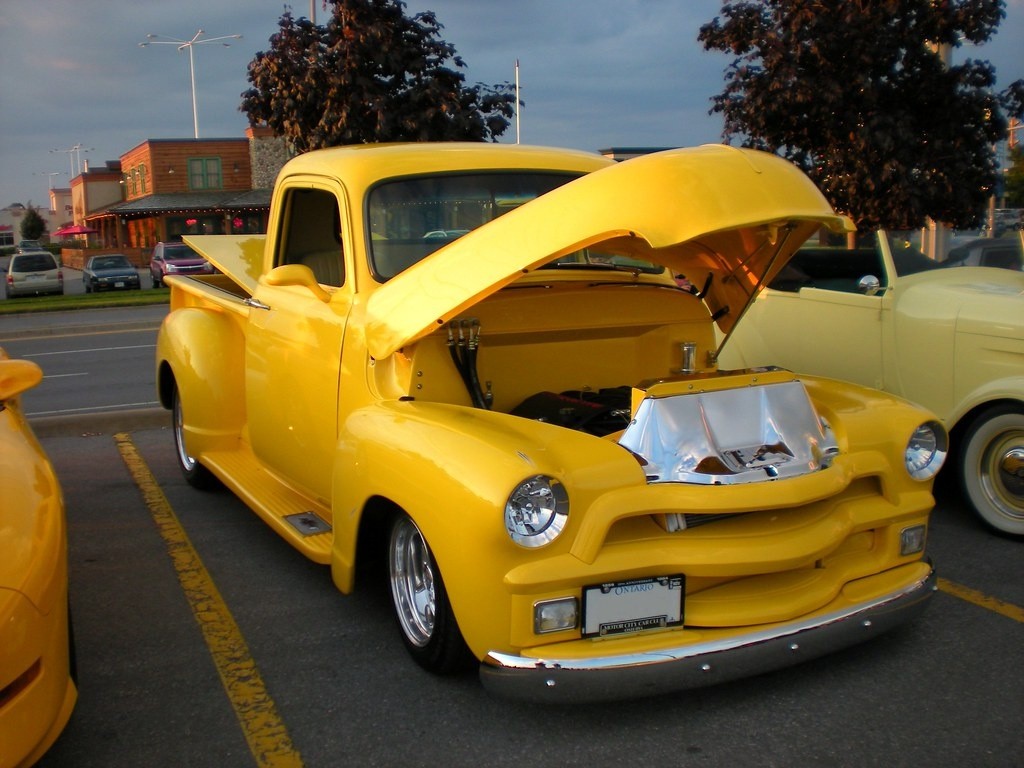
150;241;218;289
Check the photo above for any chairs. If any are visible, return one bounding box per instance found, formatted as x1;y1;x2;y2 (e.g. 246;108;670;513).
290;247;392;286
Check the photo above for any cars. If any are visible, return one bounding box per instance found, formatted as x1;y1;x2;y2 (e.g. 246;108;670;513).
0;345;81;768
0;250;64;299
989;207;1024;231
704;220;1024;543
16;239;44;256
83;253;140;293
155;143;949;704
420;228;470;240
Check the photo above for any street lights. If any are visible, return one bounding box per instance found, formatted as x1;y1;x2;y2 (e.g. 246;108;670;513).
139;29;244;137
33;169;69;191
52;142;84;179
71;144;95;177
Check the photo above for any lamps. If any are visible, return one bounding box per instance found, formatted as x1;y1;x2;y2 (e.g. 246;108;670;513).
234;163;239;172
119;173;128;183
135;164;148;174
127;169;136;180
168;163;175;173
